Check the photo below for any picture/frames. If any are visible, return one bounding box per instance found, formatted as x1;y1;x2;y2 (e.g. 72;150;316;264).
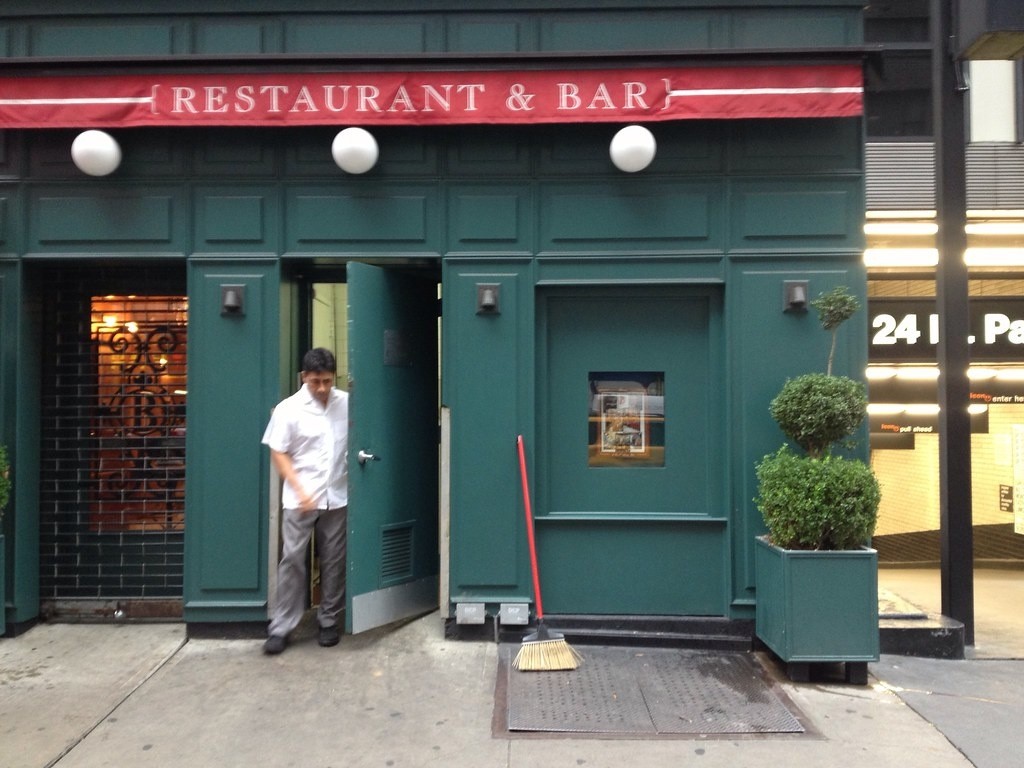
596;385;650;459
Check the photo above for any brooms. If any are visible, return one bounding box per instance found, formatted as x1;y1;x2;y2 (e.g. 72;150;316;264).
512;432;588;670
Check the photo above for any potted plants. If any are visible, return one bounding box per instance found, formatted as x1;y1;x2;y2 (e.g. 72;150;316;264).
752;288;881;684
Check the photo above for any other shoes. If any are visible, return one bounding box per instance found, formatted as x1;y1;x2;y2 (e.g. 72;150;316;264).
320;627;339;647
263;635;288;654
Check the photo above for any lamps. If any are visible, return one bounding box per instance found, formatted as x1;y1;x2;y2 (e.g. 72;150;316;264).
782;278;809;312
609;124;657;173
71;130;121;177
475;282;502;315
332;126;379;173
220;283;247;315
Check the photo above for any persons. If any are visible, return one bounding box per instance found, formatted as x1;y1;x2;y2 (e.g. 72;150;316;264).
261;347;348;655
